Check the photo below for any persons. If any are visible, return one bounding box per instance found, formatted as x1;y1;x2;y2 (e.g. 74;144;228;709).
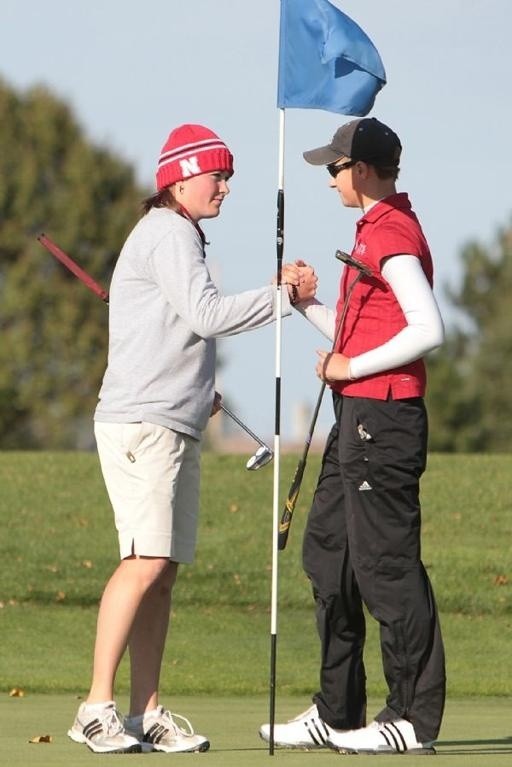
65;121;315;752
260;115;452;752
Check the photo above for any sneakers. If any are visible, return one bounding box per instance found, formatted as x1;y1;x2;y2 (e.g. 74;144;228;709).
325;713;436;756
123;704;211;753
67;700;141;754
258;704;363;749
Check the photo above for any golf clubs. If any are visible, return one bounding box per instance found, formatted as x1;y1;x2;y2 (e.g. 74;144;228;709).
38;232;273;471
278;250;375;550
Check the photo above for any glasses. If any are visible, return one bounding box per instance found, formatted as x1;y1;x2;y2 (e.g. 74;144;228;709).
325;158;361;178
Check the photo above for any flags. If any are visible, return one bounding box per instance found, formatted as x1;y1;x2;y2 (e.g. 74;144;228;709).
274;0;389;123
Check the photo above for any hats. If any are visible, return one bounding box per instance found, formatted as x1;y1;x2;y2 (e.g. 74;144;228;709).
156;123;234;194
303;118;402;169
